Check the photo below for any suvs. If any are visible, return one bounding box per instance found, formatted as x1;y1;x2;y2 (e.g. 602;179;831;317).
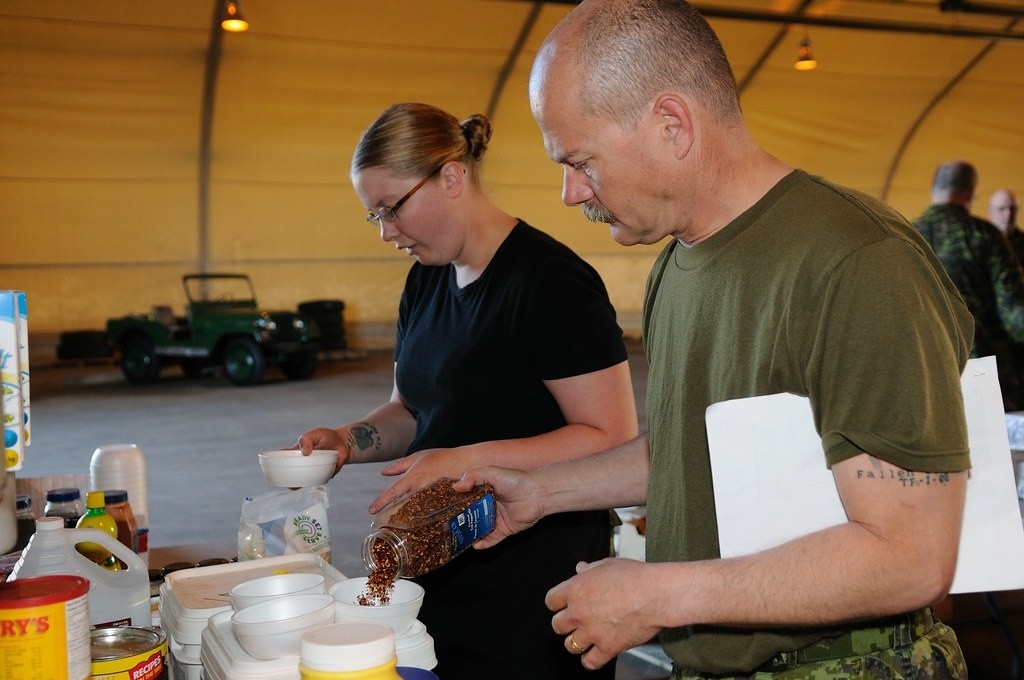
105;273;319;389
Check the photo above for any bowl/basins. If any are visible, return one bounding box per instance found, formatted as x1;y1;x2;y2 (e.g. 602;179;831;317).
327;577;425;638
230;593;335;660
257;449;339;487
89;444;149;529
228;573;325;614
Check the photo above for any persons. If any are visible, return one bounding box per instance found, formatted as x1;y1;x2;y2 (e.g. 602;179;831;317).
278;102;639;680
453;1;969;680
911;161;1024;412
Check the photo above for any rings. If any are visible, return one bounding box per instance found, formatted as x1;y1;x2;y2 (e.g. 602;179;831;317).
569;633;586;652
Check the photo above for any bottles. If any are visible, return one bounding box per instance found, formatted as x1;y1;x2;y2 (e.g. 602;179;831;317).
298;620;404;680
75;492;122;571
5;516;152;630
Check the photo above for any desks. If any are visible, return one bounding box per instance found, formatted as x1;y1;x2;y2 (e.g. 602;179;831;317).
16;475;240;570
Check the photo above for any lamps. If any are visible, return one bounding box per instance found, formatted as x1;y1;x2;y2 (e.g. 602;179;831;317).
793;36;818;71
220;0;249;32
